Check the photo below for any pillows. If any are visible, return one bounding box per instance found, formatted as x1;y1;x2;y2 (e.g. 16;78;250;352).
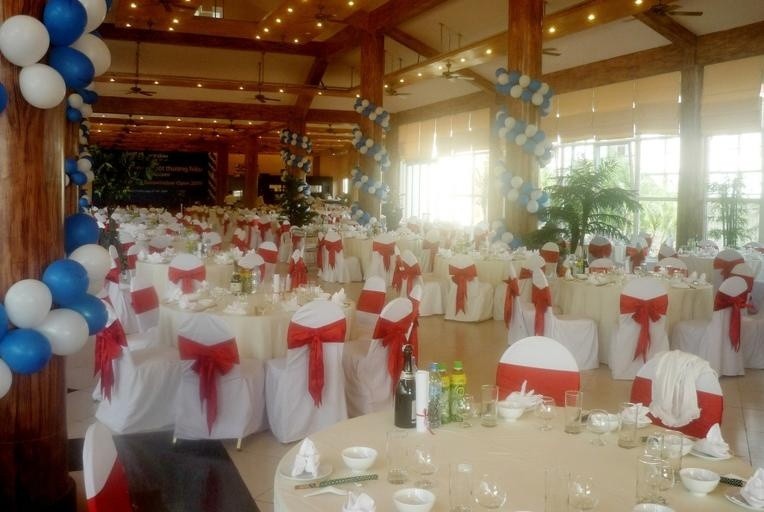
274;249;308;292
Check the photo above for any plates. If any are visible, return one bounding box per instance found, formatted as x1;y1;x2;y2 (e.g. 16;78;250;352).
724;488;764;511
279;463;333;481
223;308;246;315
689;446;732;461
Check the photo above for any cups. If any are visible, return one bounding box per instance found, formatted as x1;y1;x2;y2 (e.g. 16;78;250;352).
297;280;316;305
544;468;570;512
458;395;475;428
385;428;412;484
634;266;642;279
636;454;662;503
664;431;682;480
618;401;639;448
662;462;674;501
535;396;556;431
414;447;440;486
480;384;498;427
450;462;475;512
564;390;583;434
640;262;648;277
570;472;599;512
644;435;664;462
476;473;507;512
604;267;625;284
586;408;610;446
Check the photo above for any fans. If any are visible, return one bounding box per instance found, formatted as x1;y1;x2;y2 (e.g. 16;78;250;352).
120;87;157;96
624;0;703;22
240;90;281;103
541;47;561;56
385;88;413;96
423;61;476;81
336;86;359;92
297;7;346;23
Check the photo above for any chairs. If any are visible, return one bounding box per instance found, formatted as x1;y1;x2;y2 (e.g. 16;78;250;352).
234;215;249;234
127;245;146;279
320;213;324;223
184;207;191;218
256;253;264;285
654;257;688;277
106;260;136;333
278;220;292;263
713;250;744;293
197;207;202;223
744;242;762;251
251;208;257;215
149;208;155;216
392;250;448;316
93;307;180;434
230;227;242;248
92;292;163;402
350;277;385;346
241;253;265;287
408;216;417;232
291;227;305;252
265;301;346;443
587;236;615;265
222;214;233;237
324;231;363;283
265;208;271;214
588;258;613;273
658;243;678;262
255;241;278;281
183;215;191;226
418;231;440;273
169;253;206;295
108;245;122;273
83;423;131;512
644;233;652;248
727;263;754;292
540;241;559;276
115;220;120;232
316;231;325;277
609;279;669;380
248;215;259;249
105;206;108;216
532;267;599;371
205;232;222;255
625;241;641;274
216;208;223;230
343;299;413;416
408;285;422;368
669;276;751;377
364;235;401;285
149;238;169;255
257;215;275;246
328;218;333;224
176;213;183;224
336;216;340;223
237;230;249;253
490;240;510;254
119;229;136;260
172;313;265;451
504;263;536;345
166;225;178;240
496;336;581;406
203;207;211;223
130;277;158;331
258;207;261;215
637;238;648;261
202;223;212;241
445;254;494;322
181;203;183;213
104;219;110;234
493;256;545;320
193;220;201;235
191;206;197;220
630;351;723;438
698;240;718;249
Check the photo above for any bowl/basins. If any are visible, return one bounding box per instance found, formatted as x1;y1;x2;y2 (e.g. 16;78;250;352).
342;446;378;474
679;467;721;496
498;402;524;422
608;414;619;433
392;487;436;512
683;437;694;457
632;503;675;512
576;273;588;280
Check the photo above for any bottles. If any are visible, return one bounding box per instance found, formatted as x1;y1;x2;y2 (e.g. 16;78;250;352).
251;266;259;295
583;250;588;274
231;260;243;295
577;257;584;274
428;363;442;429
451;361;467;423
438;363;451;424
395;346;416;429
